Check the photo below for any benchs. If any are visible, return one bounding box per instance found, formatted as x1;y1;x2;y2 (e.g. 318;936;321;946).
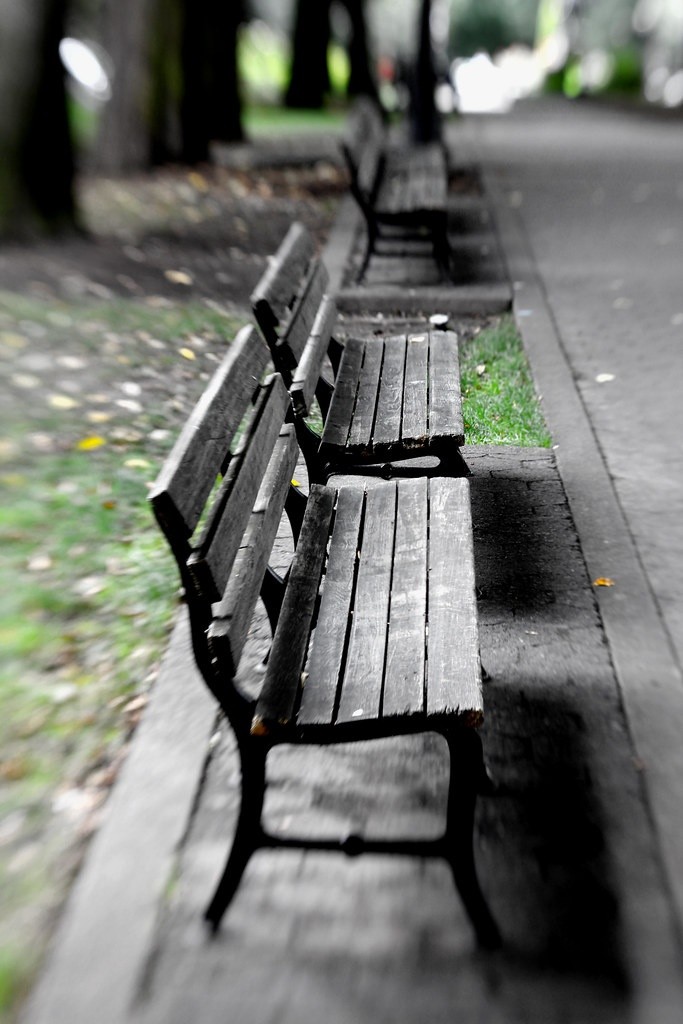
149;323;513;966
250;224;470;489
338;98;463;285
